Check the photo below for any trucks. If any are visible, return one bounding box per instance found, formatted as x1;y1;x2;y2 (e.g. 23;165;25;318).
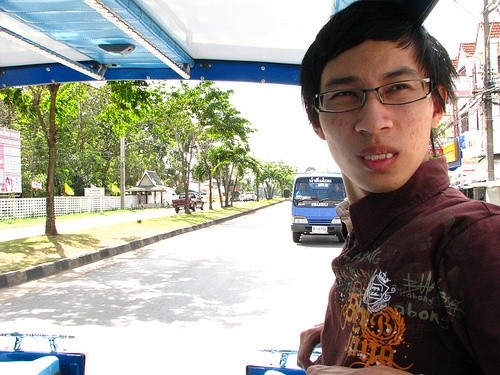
283;172;347;243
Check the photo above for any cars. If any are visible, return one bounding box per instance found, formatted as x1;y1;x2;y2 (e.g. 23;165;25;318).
243;192;257;201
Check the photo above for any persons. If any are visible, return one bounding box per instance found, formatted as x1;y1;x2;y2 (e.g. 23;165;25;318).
296;14;500;375
329;183;343;197
297;183;311;196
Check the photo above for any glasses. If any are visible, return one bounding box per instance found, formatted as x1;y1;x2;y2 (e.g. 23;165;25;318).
314;78;440;114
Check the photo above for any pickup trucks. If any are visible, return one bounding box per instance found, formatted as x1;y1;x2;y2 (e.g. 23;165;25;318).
173;192;204;213
